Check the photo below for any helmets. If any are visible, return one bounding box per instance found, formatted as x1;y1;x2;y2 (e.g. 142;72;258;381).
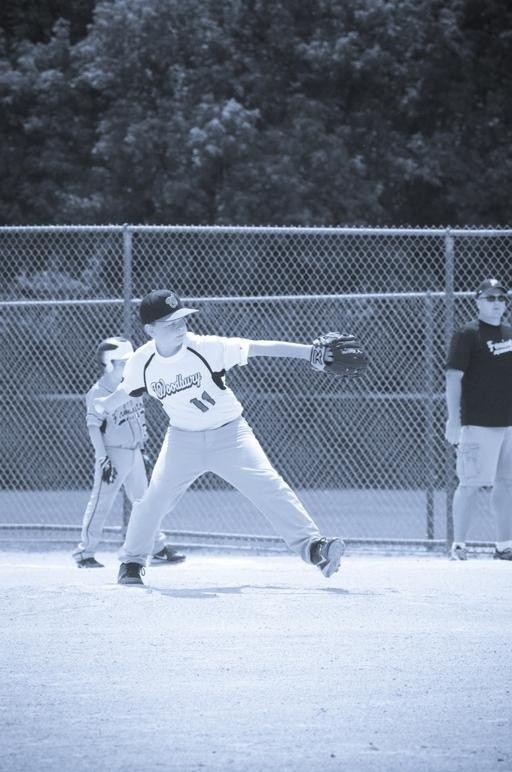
96;336;134;373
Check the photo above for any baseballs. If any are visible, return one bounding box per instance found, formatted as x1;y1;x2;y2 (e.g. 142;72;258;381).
93;403;106;414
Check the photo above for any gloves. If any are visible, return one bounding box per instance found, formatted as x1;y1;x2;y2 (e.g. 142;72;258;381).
99;455;118;485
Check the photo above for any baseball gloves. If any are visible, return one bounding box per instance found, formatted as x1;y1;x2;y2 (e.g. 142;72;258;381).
310;331;368;379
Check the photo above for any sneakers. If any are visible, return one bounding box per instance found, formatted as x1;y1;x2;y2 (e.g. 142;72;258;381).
117;562;145;586
310;536;345;578
492;546;512;561
152;545;187;564
77;558;105;569
448;544;468;561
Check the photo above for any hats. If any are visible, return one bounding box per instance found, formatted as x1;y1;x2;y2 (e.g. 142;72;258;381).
139;289;200;324
476;278;507;297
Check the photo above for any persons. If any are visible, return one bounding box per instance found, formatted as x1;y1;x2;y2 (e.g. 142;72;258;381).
444;279;512;563
78;339;186;567
92;290;368;586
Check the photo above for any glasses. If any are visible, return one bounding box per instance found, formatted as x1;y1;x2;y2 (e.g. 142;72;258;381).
478;295;506;303
